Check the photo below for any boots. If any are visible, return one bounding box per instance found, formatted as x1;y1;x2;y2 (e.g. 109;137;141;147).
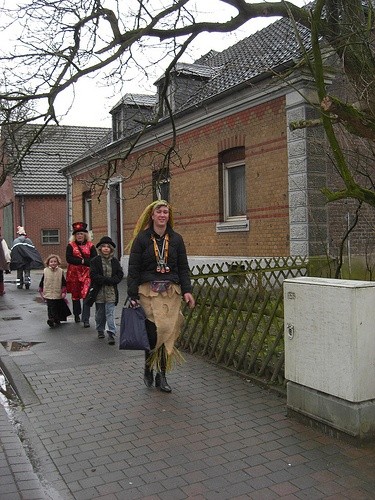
0;282;5;296
155;343;173;393
144;345;155;389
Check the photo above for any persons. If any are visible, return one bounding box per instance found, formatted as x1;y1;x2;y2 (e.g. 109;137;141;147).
38;254;72;327
85;236;124;344
119;199;194;392
65;221;98;327
0;227;12;296
10;226;45;289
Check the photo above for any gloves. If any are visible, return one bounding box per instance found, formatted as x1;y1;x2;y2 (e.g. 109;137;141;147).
5;269;11;274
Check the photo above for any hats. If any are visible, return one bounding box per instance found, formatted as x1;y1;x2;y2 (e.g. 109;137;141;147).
96;236;116;249
16;226;26;236
72;222;88;235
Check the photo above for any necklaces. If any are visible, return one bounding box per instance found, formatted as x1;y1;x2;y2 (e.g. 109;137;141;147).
152;233;170;273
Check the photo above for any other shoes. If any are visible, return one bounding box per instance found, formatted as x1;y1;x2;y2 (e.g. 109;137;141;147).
84;320;91;327
47;318;60;328
108;334;116;343
74;315;81;322
97;330;105;338
18;286;22;289
26;284;29;289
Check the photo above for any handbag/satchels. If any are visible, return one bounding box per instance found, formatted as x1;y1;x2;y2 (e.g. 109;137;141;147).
118;298;151;353
